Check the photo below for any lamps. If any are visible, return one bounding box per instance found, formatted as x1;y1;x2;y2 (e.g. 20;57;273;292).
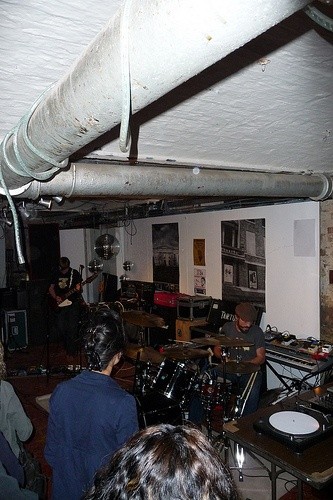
21;196;63;218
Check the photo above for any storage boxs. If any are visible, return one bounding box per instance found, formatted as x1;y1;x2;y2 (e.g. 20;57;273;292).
177;296;212;320
0;308;28;351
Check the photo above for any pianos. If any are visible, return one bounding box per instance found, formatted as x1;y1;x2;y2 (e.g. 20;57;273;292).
263;330;333;393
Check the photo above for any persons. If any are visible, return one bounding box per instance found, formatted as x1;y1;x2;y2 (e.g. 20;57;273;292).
42;312;139;500
213;304;265;414
0;342;33;458
49;257;86;308
81;423;243;500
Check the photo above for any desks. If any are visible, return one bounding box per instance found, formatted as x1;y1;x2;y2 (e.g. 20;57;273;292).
222;382;333;500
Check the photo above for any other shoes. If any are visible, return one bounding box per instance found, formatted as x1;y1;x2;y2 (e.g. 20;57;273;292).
66;354;74;362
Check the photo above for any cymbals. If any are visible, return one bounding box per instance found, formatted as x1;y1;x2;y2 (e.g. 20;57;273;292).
218;361;260;375
158;344;211;359
191;336;257;346
120;310;168;328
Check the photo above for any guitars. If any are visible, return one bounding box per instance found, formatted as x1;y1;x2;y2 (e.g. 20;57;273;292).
53;274;99;307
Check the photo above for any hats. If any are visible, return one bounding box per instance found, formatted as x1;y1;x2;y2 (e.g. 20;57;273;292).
235;303;258;321
58;256;70;271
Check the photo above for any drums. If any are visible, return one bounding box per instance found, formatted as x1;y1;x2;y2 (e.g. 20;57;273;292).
150;358;202;405
201;375;231;404
202;391;238;441
